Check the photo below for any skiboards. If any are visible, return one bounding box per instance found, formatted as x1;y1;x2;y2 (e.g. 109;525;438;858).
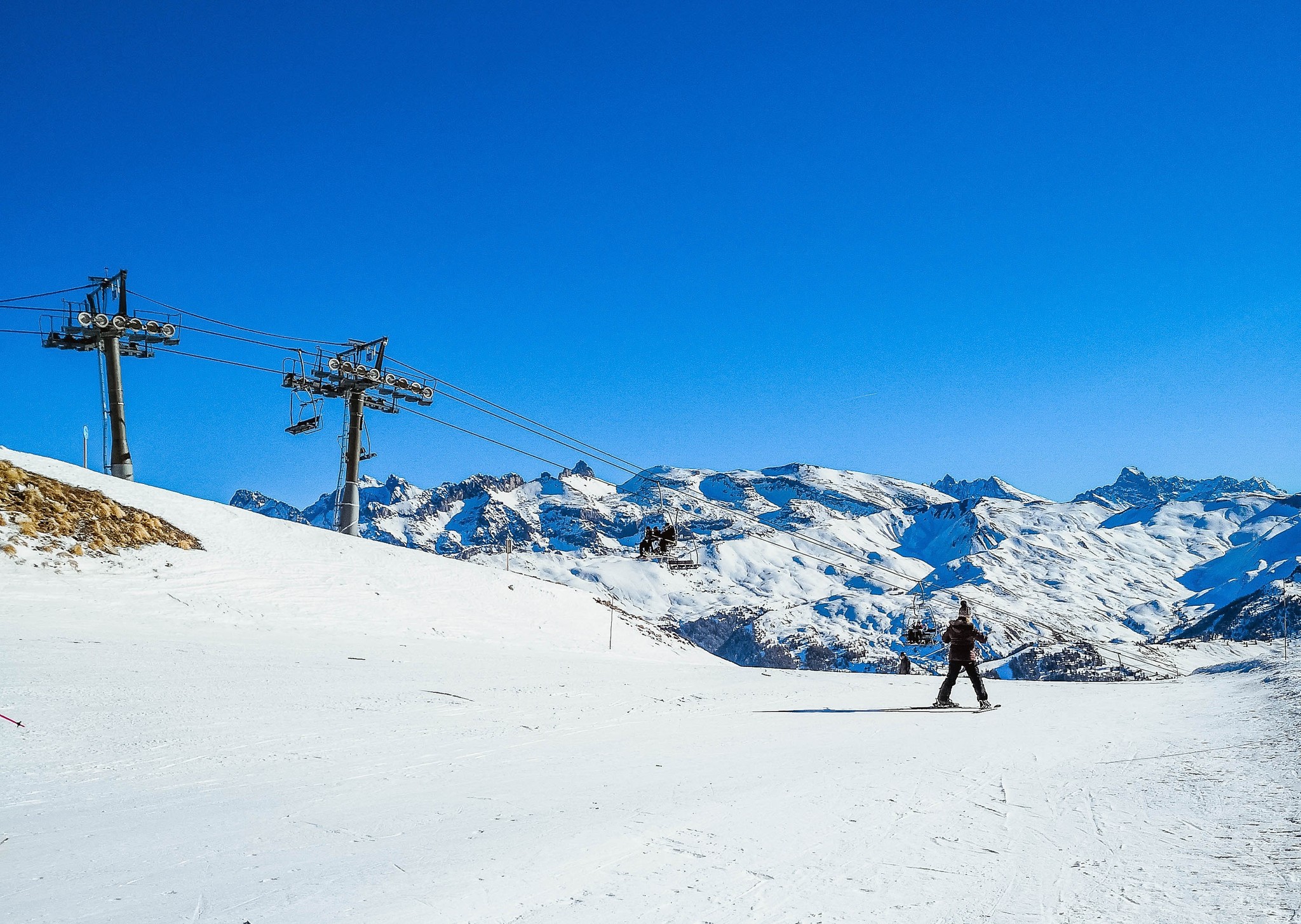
909;699;1002;713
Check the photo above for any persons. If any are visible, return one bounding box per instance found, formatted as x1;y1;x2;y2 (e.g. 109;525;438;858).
636;522;675;558
937;600;991;711
898;651;911;674
908;619;928;646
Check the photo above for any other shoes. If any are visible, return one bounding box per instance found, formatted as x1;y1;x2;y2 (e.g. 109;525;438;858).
983;700;991;708
939;699;959;706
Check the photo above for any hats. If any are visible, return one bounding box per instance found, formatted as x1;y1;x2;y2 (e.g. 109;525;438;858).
959;600;973;617
